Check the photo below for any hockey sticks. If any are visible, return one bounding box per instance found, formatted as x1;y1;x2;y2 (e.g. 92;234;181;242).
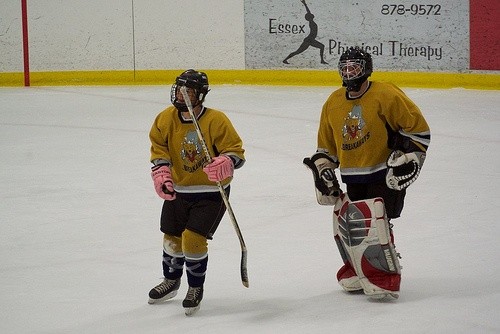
179;87;249;288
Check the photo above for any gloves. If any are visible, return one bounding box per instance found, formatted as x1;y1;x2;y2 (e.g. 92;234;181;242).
203;155;234;182
151;163;176;201
303;152;343;206
385;149;426;191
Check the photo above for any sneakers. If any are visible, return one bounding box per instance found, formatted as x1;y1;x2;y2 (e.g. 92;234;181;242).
148;278;181;304
182;285;203;316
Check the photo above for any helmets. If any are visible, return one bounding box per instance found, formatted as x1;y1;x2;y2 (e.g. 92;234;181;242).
338;48;373;92
170;69;211;112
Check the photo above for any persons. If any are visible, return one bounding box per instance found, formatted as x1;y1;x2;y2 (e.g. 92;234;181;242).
303;47;431;301
147;69;247;317
